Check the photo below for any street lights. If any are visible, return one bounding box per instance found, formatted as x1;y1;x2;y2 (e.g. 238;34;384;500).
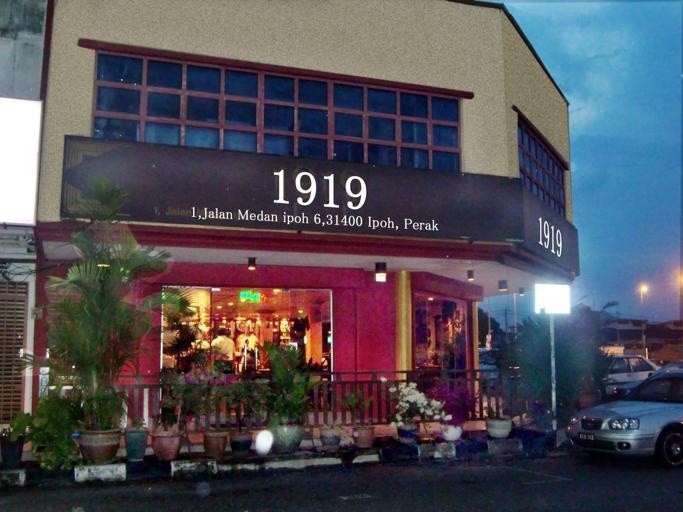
534;283;571;430
641;286;648;356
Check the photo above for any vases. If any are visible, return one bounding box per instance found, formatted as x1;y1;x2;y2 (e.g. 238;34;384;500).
390;422;466;443
200;367;228;458
125;409;150;462
52;254;124;458
150;304;198;460
230;371;252;450
258;342;313;455
320;376;340;453
346;389;374;448
340;435;358;462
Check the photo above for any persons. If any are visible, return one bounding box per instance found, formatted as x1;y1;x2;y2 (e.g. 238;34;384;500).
210;326;235;372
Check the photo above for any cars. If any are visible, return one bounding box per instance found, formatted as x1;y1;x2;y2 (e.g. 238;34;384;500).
565;355;683;469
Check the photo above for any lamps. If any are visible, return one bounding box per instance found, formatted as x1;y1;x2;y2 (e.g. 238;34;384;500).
375;262;387;283
519;288;525;296
498;280;509;292
246;257;257;271
466;270;475;281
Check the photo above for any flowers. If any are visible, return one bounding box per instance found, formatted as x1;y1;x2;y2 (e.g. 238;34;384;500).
380;374;478;424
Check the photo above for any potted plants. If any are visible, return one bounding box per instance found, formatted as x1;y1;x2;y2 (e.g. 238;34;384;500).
485;386;513;441
